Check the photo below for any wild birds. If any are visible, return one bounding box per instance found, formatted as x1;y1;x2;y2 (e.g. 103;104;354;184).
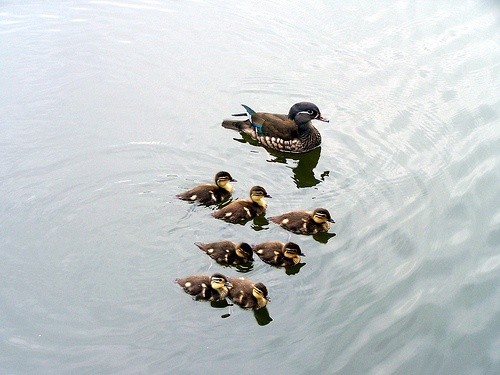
266;208;335;235
211;186;272;224
252;242;306;267
193;240;254;265
174;273;233;302
176;170;238;206
224;277;272;310
221;101;329;153
